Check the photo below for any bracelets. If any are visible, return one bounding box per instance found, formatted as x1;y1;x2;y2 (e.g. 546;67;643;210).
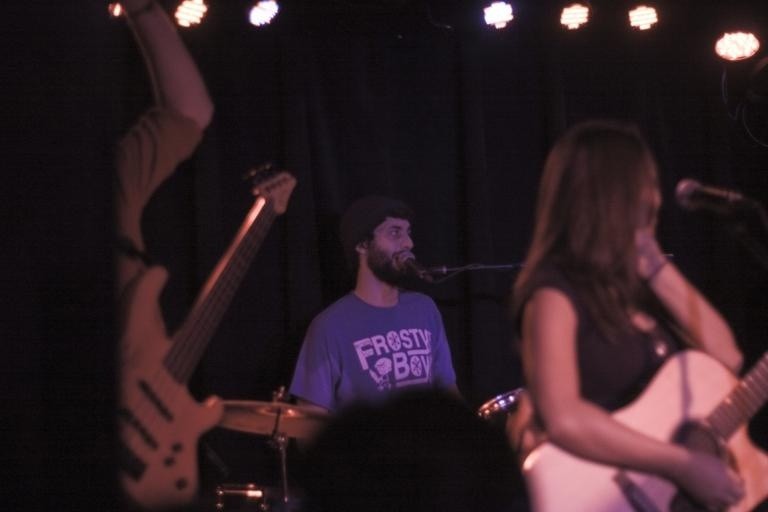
126;2;156;17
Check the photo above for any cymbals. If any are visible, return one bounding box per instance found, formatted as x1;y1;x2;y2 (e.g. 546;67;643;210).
217;402;329;439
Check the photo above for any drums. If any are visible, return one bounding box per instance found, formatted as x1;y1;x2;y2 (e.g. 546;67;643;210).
214;484;298;511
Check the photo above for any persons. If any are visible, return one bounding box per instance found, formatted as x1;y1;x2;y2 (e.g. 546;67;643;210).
0;1;217;512
287;195;465;414
510;123;747;512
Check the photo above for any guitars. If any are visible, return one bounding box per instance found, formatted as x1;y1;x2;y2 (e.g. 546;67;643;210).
522;349;767;512
113;162;296;508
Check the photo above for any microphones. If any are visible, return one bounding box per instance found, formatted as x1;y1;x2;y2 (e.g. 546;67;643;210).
396;250;436;288
675;179;768;222
